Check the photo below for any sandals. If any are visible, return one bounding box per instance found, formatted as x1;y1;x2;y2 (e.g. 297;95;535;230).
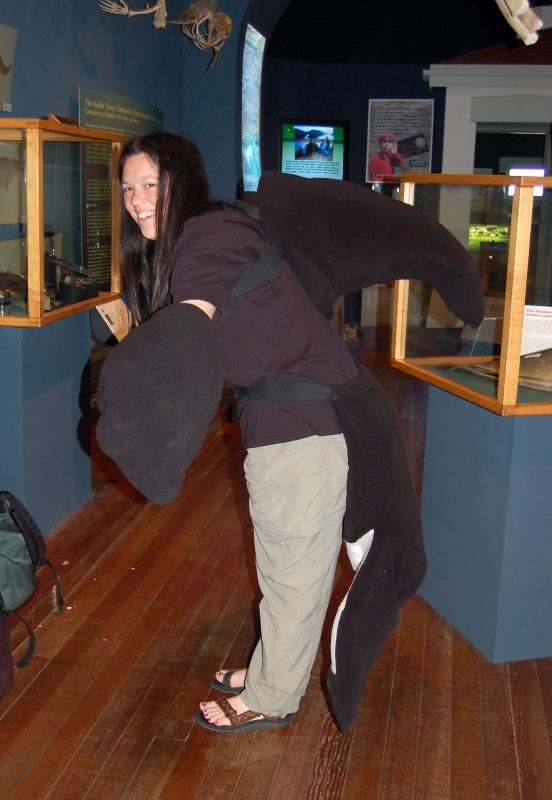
211;668;247;696
196;690;293;732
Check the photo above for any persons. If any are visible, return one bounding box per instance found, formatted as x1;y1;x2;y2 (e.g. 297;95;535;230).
367;133;404;182
91;134;352;733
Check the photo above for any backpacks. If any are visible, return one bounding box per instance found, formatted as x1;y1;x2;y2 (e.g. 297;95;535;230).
0;489;49;612
